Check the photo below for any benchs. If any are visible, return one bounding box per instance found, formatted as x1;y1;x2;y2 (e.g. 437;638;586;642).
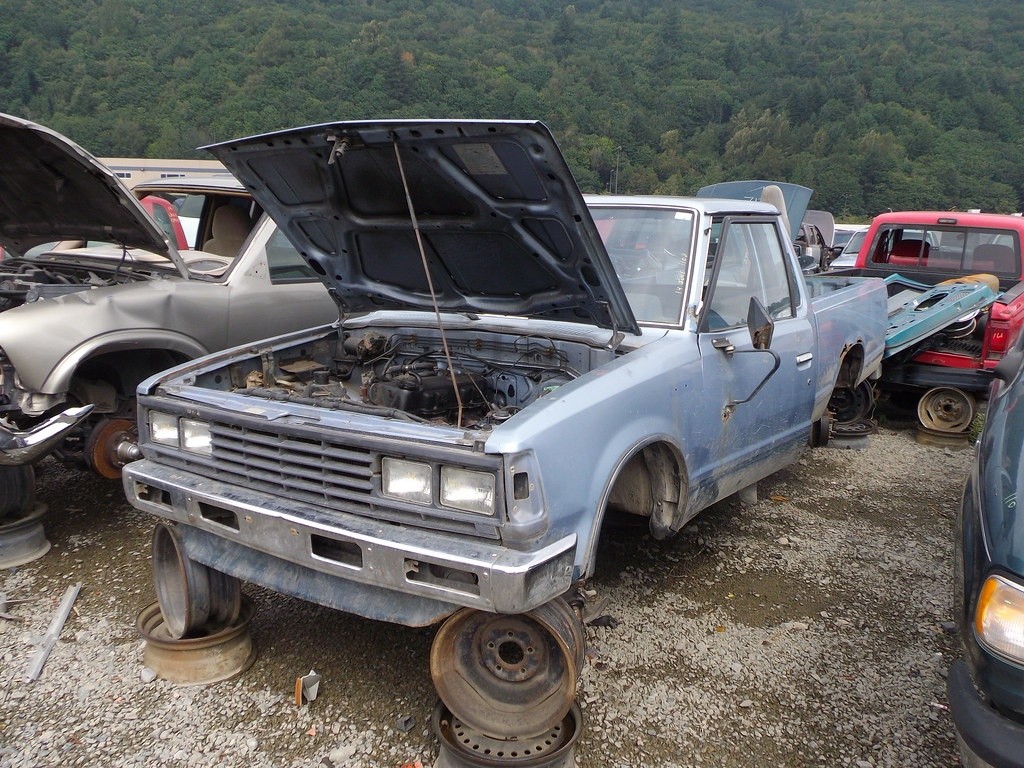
891;255;995;271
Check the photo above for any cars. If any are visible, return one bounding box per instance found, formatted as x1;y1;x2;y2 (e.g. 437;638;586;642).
695;174;1022;406
941;332;1024;768
1;111;342;510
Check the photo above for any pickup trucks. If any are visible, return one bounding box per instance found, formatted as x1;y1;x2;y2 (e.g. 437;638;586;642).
116;117;893;621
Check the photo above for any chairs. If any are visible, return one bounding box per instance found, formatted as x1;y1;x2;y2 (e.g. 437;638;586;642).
204;208;247;259
886;240;932;265
973;243;1015;274
